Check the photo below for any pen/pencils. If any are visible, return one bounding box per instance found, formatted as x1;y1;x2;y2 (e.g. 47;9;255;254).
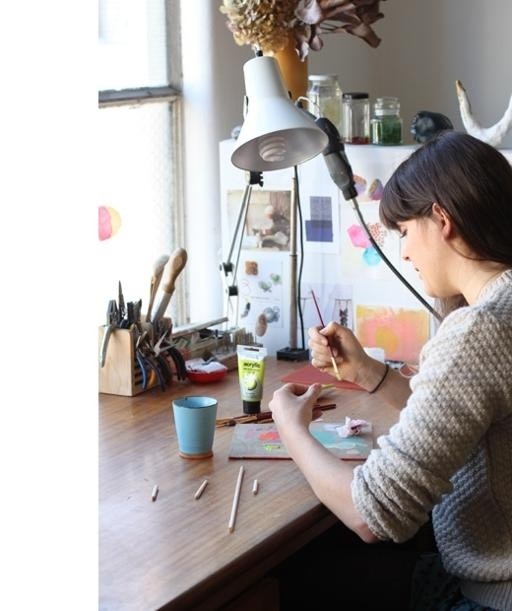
228;466;244;533
194;480;208;500
152;486;159;501
252;480;258;494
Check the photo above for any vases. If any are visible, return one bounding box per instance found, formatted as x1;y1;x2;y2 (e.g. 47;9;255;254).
261;27;308;111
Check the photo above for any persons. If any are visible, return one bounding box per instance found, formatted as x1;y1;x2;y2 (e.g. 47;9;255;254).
268;130;512;611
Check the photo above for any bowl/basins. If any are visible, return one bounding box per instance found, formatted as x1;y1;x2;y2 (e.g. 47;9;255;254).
184;370;225;384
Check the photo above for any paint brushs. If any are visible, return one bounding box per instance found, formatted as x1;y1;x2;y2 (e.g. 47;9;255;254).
310;290;342;382
215;404;336;427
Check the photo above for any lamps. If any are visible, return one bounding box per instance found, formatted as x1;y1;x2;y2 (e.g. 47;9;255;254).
230;57;331;362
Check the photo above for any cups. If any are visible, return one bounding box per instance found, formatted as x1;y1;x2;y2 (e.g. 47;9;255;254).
171;396;219;459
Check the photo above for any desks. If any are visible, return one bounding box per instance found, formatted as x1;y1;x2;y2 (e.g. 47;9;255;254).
98;355;420;611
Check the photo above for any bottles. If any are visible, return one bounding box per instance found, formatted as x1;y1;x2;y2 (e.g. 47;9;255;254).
371;97;403;146
308;74;370;144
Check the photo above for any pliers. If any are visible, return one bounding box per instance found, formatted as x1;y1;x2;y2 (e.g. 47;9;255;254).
99;281;186;392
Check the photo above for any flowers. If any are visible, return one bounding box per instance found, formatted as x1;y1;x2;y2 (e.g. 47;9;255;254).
219;0;385;63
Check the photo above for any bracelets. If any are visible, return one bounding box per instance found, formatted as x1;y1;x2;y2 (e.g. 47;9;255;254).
367;361;390;395
